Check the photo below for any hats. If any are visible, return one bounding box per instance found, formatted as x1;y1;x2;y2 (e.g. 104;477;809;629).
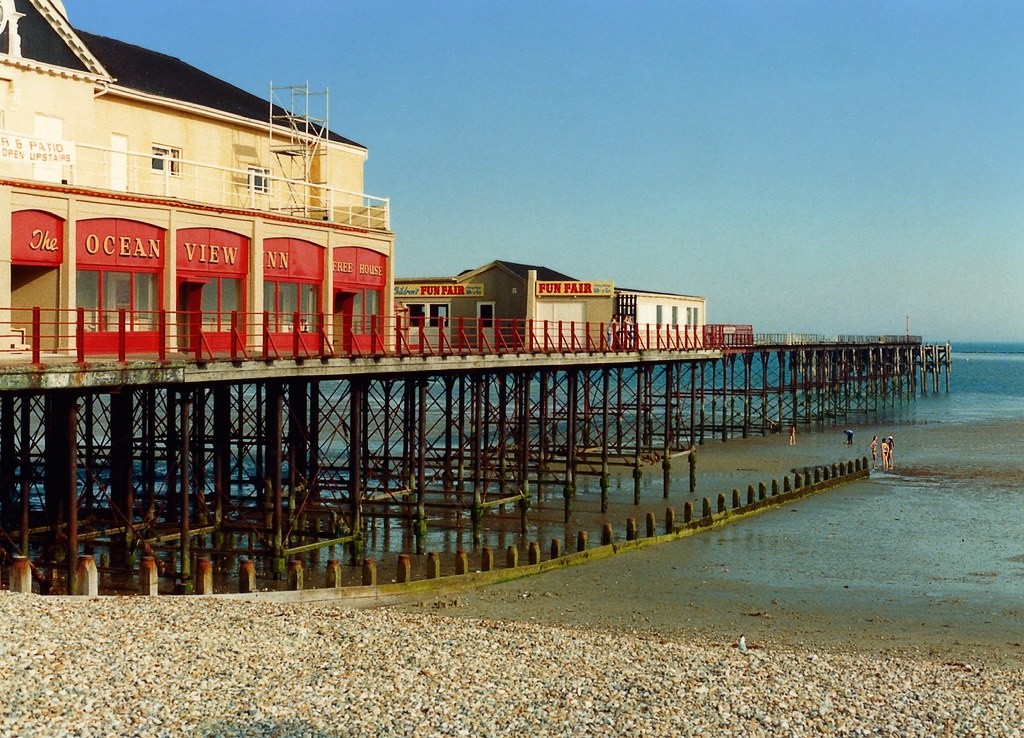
888;435;893;439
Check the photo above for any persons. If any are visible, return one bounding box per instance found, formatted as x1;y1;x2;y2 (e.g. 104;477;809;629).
880;435;895;472
769;422;782;436
605;315;620;351
623;314;635;348
789;425;797;446
844;429;854;445
870;435;878;470
